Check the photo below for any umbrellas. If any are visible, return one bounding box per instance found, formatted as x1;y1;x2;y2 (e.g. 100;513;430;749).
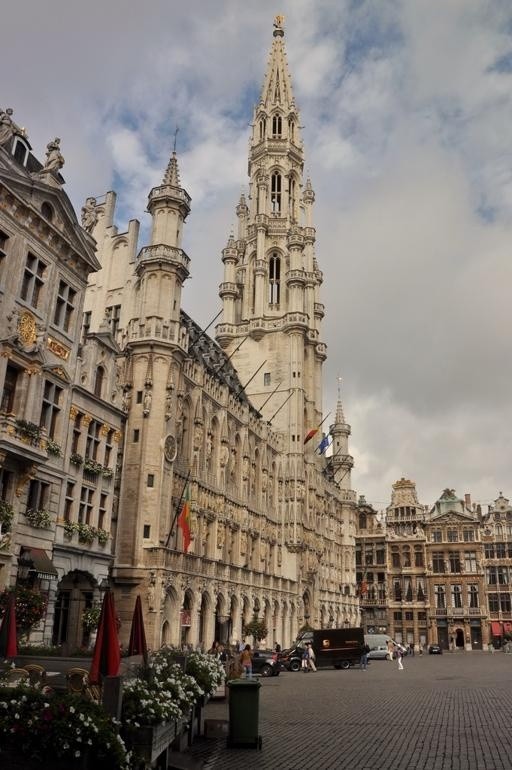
89;590;121;687
128;594;149;667
0;590;21;664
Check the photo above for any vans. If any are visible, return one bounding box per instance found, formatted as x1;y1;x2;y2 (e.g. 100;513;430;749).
282;627;366;671
363;634;407;658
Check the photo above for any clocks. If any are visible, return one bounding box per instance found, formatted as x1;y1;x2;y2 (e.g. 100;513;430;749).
161;434;179;465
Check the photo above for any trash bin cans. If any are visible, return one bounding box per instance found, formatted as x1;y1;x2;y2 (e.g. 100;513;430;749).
226;677;262;751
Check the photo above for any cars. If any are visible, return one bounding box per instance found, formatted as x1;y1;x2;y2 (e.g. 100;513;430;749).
367;646;392;661
221;649;282;676
428;644;442;655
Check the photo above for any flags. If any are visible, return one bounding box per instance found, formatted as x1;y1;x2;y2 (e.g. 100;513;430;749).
360;576;367;594
177;484;192;556
319;436;329;455
304;424;318;445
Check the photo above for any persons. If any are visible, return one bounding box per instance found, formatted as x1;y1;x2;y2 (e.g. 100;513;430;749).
301;645;309;670
8;308;22;336
360;642;371;671
396;646;405;670
35;324;47;353
275;642;281;652
0;107;16;147
207;639;254;681
305;644;317;673
385;639;424;661
47;137;63;173
